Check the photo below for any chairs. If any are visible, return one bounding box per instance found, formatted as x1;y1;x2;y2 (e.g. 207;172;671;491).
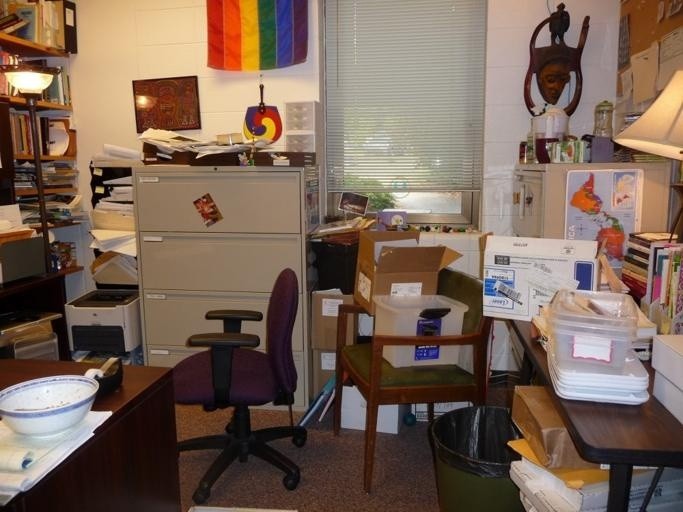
333;266;492;491
174;267;307;506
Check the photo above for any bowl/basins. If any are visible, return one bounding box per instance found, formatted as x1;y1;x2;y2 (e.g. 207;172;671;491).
0;375;98;438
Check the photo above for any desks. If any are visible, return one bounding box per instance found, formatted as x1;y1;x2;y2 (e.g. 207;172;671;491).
504;319;683;512
0;356;180;512
309;240;358;295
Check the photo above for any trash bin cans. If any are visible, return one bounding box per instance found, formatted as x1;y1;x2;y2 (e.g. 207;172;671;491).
428;406;526;512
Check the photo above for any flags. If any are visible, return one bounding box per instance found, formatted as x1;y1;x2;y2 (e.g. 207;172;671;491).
205;0;310;72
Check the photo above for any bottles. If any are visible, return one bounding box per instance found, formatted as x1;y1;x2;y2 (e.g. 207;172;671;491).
592;100;613;137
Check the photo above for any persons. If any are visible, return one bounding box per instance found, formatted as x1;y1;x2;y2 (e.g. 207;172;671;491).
536;54;571;104
549;3;570;46
143;79;194;131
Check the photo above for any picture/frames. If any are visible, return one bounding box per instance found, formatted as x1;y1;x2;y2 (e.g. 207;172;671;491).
132;75;201;133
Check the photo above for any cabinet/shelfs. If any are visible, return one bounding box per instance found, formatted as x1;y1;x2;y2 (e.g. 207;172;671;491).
130;165;311;412
511;160;674;291
0;32;85;362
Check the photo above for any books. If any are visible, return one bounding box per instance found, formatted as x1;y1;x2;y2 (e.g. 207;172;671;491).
1;1;82;220
621;232;683;319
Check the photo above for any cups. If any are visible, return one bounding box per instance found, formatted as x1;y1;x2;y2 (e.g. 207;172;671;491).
531;112;570;161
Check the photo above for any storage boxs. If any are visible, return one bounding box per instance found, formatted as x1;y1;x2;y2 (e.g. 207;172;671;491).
410;401;473;422
482;235;609;322
309;350;336;401
340;385;411;435
309;292;354;350
353;229;463;317
64;289;141;354
371;294;470;369
8;331;59;360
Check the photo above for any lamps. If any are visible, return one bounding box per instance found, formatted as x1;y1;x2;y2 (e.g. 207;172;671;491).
0;63;62;313
610;67;682;161
